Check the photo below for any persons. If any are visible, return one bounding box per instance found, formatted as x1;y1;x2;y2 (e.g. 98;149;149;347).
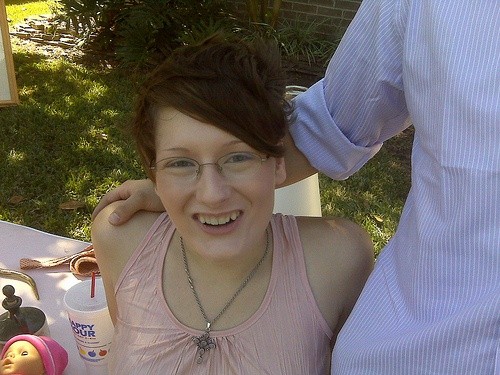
91;0;500;375
90;31;376;375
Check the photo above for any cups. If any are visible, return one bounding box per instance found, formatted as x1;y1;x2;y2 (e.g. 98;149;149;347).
63;279;114;366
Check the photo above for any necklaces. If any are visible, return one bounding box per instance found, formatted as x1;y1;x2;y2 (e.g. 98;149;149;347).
180;229;269;364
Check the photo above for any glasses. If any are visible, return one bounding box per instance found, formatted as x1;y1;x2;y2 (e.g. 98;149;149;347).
142;151;272;187
